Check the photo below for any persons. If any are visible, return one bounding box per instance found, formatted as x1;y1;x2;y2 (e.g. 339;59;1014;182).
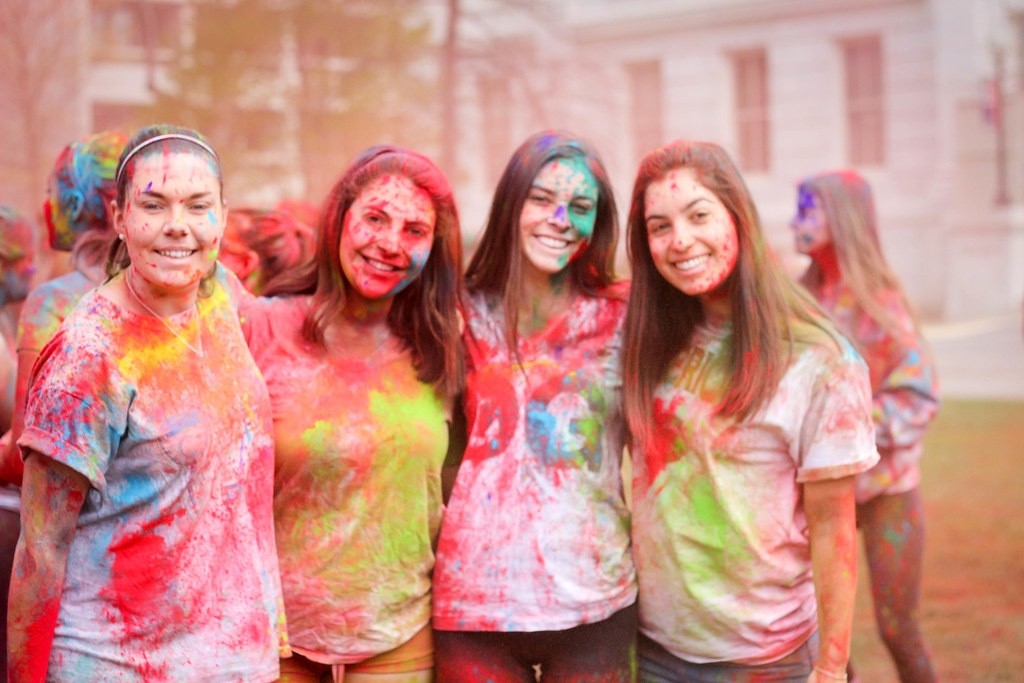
787;172;936;683
0;123;880;683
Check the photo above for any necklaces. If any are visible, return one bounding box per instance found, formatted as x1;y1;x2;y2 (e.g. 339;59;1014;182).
124;269;206;358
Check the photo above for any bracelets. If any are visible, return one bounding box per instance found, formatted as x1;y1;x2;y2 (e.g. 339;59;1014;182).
811;664;848;680
